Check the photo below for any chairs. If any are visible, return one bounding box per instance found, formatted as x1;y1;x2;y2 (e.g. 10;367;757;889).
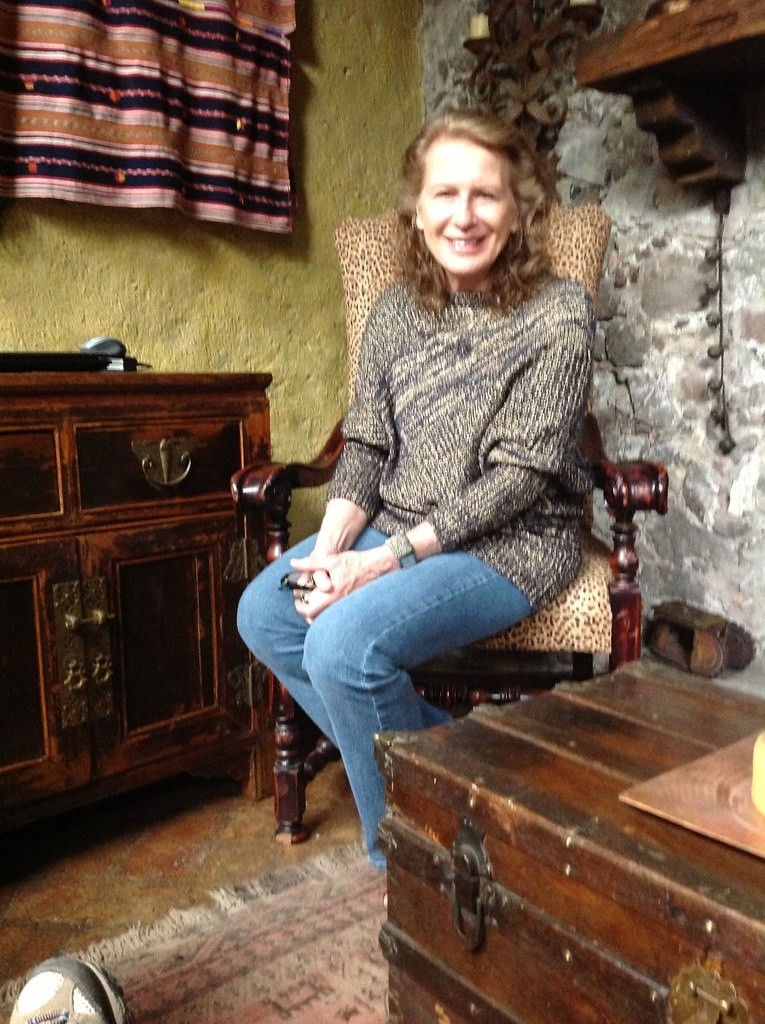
228;201;667;845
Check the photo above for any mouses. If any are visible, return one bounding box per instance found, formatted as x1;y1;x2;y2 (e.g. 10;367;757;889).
80;337;126;354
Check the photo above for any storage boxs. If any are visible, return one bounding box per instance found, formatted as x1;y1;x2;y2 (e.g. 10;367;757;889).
369;654;764;1024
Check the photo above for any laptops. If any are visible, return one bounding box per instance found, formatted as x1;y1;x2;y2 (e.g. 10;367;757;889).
0;353;137;373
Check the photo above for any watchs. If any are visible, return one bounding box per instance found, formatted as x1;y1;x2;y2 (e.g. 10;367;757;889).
385;532;418;569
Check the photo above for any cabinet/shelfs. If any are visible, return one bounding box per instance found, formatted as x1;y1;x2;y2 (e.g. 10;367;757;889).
0;369;277;855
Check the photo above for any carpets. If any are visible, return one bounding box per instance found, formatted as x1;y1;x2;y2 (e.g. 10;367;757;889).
0;839;390;1024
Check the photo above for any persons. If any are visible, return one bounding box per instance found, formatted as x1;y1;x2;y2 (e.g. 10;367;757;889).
236;106;593;869
